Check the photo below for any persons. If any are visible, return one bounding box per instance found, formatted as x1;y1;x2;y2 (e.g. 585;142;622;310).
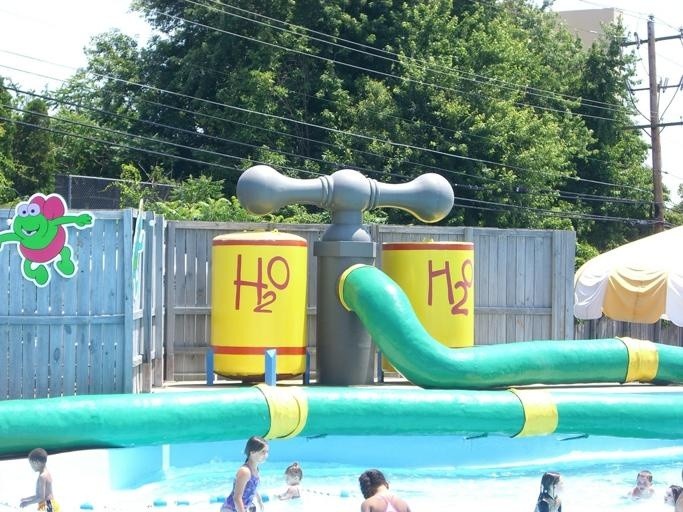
276;462;303;501
663;486;680;507
533;471;564;512
627;470;654;498
18;447;58;512
358;469;410;512
218;435;267;512
